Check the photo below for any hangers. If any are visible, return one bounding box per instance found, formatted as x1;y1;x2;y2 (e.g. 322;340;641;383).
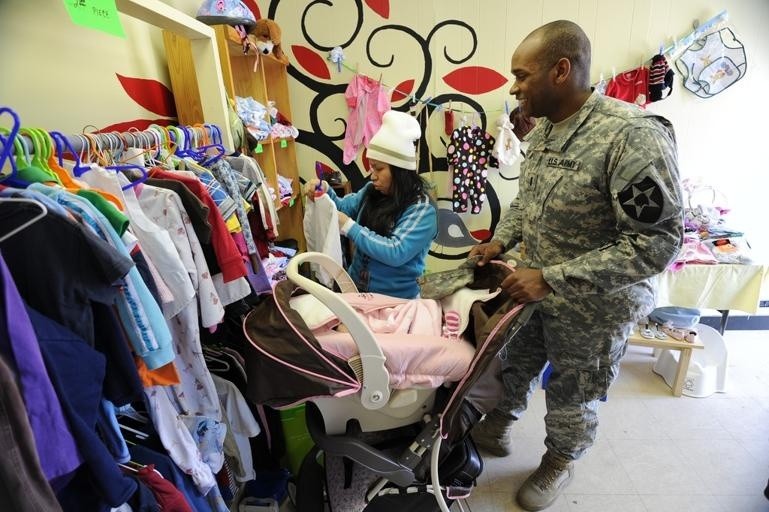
0;105;226;245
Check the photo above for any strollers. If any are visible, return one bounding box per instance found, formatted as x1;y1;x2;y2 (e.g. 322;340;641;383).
239;251;535;512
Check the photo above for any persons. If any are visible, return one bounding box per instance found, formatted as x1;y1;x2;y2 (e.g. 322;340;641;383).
302;109;439;300
468;19;686;511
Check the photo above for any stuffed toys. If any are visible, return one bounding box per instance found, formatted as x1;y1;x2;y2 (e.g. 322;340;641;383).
249;17;289;66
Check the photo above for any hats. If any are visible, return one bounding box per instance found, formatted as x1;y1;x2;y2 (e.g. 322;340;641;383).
648;54;674;102
366;110;422;171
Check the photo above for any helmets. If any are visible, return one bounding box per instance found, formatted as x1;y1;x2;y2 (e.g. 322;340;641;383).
195;0;257;34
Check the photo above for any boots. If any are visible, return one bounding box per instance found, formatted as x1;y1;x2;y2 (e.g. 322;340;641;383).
516;444;574;512
468;411;513;457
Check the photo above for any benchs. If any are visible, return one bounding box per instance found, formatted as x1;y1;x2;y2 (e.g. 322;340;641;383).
626;320;704;397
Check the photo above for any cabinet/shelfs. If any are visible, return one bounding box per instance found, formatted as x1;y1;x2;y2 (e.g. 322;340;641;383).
162;21;312;297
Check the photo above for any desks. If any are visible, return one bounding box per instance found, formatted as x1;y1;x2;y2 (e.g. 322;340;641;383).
661;258;767;336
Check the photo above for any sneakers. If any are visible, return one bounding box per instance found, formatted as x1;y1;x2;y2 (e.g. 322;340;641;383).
639;325;696;343
